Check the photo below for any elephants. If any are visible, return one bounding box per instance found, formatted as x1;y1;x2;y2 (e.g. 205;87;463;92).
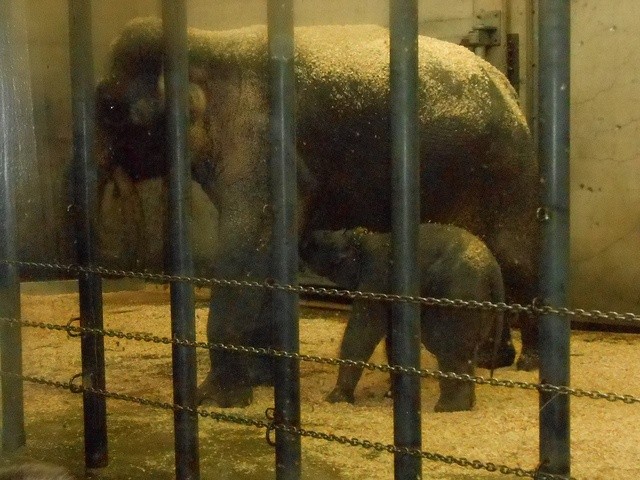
60;17;542;406
298;222;506;413
